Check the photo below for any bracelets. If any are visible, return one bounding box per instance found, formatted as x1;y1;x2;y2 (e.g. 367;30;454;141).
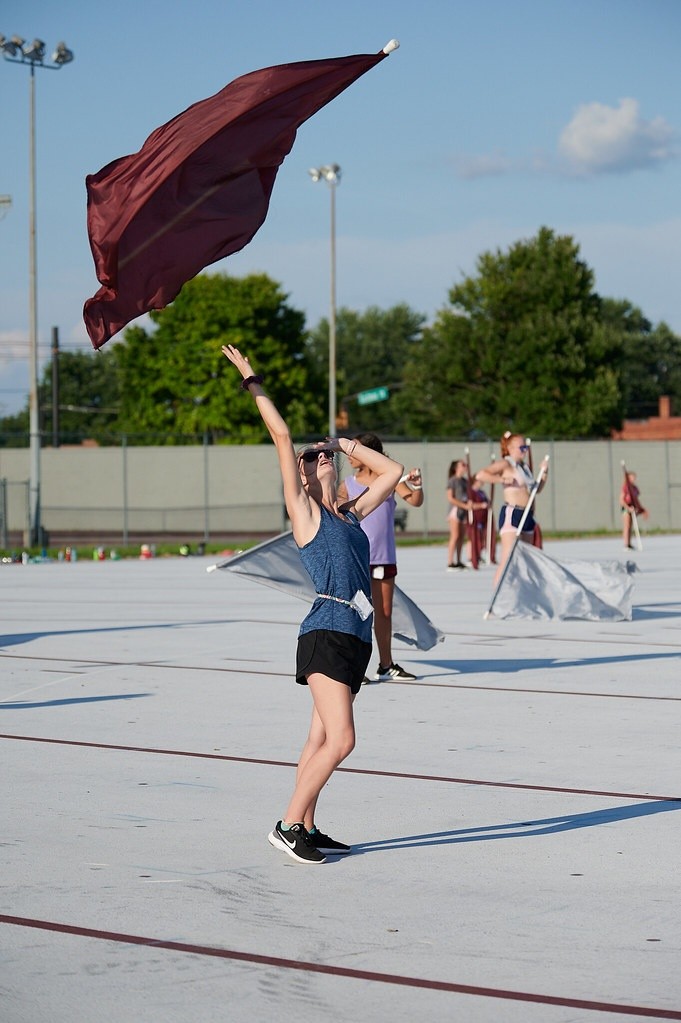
347;440;356;454
243;375;263;390
412;485;422;490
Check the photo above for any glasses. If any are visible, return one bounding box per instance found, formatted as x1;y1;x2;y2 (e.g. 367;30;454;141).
519;446;528;452
298;449;334;468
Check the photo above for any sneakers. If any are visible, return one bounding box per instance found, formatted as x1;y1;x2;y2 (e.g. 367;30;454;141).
375;662;416;680
360;676;369;684
267;821;326;864
308;825;351;854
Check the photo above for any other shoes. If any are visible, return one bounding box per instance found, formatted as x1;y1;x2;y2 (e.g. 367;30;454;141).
467;559;486;568
447;563;468;572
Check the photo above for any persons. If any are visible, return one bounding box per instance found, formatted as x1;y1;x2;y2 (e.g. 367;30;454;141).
337;433;423;685
475;434;548;586
221;344;404;864
620;472;646;550
445;460;473;570
468;475;489;563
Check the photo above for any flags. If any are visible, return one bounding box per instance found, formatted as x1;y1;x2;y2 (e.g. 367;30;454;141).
489;541;633;623
84;47;388;347
624;469;645;515
215;530;443;650
465;450;501;567
